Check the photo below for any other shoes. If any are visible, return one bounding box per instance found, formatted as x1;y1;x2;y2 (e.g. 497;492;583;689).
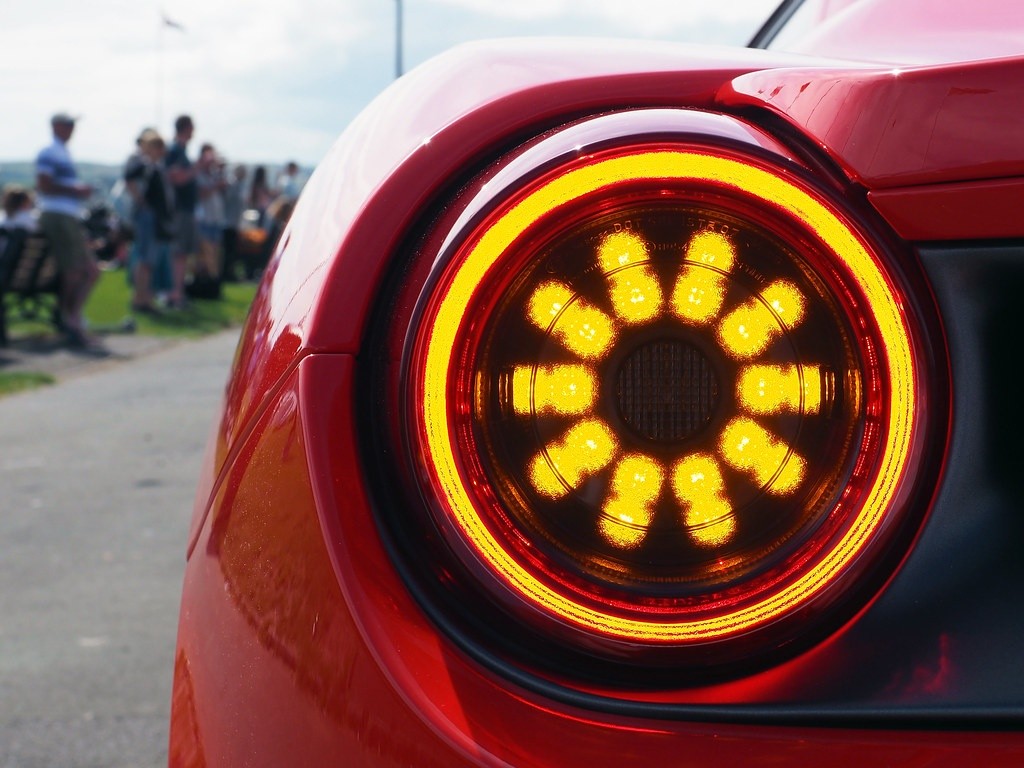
167;299;184;309
51;306;85;335
130;301;160;315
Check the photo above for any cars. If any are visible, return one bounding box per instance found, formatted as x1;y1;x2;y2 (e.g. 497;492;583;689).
168;1;1024;768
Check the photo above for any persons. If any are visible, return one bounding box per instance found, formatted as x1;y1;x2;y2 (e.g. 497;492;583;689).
0;112;303;358
